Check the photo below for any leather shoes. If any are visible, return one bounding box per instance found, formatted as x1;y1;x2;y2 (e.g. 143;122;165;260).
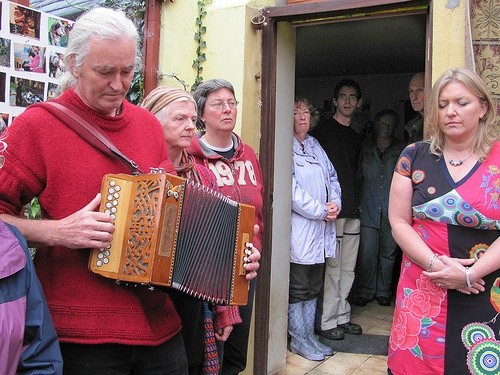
376;296;391;306
354;296;368;307
319;328;345;340
337;322;362;335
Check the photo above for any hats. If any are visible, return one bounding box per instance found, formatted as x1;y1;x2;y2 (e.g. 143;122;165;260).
141;86;197;116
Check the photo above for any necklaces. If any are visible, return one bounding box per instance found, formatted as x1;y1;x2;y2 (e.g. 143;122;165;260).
445;148;472;166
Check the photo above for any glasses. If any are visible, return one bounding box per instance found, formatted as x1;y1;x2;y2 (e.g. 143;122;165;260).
380;123;395;130
203;100;240;109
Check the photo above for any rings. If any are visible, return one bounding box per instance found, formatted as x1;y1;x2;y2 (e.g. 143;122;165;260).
438;282;441;287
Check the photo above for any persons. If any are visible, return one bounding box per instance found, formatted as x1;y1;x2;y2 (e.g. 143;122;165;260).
0;8;262;375
0;220;63;375
28;51;40;71
50;22;73;48
388;67;500;375
308;79;363;340
140;86;243;375
287;92;341;361
14;81;24;108
14;6;29;37
404;72;424;146
350;109;408;307
192;79;265;375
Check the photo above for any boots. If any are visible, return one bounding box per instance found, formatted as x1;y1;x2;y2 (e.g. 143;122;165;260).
288;300;325;360
302;297;335;355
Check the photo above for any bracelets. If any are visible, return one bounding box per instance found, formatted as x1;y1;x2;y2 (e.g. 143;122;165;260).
466;267;473;287
427;253;438;272
